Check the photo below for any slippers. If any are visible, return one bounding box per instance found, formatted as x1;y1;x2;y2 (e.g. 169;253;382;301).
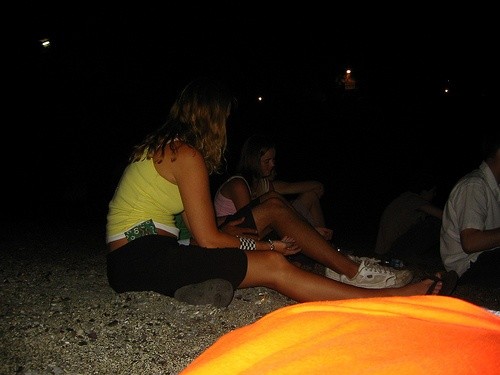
174;278;234;307
423;269;459;295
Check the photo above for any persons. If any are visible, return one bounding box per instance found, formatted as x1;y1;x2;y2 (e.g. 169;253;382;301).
213;134;443;289
440;137;500;288
106;74;457;303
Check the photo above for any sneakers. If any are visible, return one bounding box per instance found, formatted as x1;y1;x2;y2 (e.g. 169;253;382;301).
324;254;414;289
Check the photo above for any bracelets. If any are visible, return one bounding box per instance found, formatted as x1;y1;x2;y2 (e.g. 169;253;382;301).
269;239;275;251
